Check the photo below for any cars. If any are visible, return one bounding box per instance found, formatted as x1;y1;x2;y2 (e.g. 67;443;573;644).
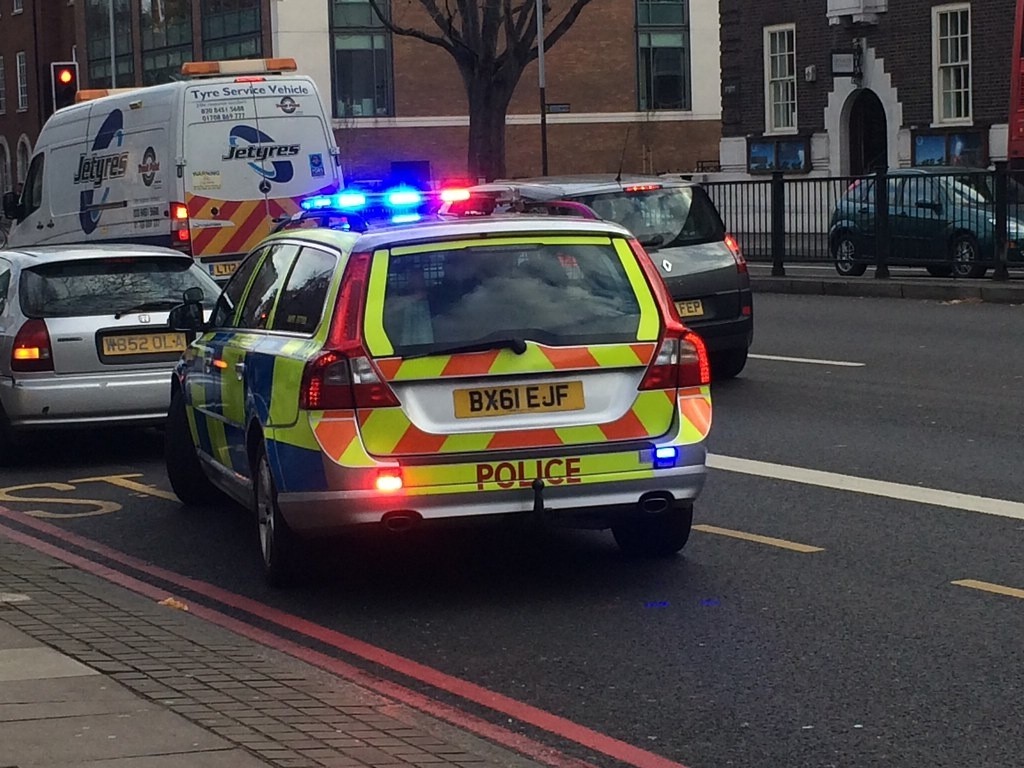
163;184;712;586
0;242;234;444
438;125;754;380
828;166;1024;279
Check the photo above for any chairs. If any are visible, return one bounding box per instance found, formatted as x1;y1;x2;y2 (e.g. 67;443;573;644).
389;197;655;319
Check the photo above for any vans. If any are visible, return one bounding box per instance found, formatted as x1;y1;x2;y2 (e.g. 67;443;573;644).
1;56;347;291
